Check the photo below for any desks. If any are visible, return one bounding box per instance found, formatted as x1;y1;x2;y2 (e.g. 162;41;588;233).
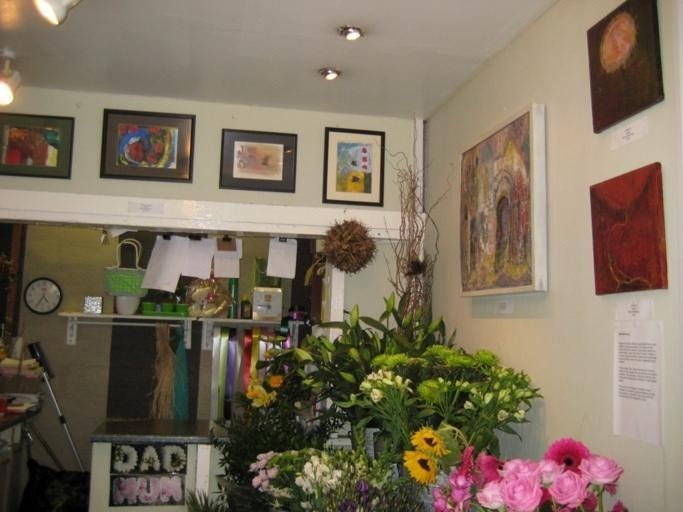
89;420;237;511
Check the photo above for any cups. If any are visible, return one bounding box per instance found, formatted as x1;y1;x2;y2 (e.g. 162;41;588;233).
241;294;251;319
175;303;190;313
141;302;156;311
160;303;174;313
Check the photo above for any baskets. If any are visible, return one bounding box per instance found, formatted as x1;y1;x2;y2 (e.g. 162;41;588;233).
102;267;147;296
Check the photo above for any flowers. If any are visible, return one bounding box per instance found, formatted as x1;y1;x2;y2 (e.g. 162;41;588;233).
248;292;628;512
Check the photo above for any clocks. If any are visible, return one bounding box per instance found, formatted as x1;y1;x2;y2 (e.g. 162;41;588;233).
23;278;63;314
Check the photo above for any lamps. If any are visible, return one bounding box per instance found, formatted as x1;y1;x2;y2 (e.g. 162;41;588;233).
32;0;81;26
0;48;22;106
337;26;362;41
318;68;339;80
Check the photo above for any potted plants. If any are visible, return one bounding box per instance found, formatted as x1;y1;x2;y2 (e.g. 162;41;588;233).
209;365;349;512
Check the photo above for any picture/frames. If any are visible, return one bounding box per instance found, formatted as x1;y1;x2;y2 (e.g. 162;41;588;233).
457;101;548;297
100;109;195;183
218;129;297;192
0;113;75;178
322;127;385;206
